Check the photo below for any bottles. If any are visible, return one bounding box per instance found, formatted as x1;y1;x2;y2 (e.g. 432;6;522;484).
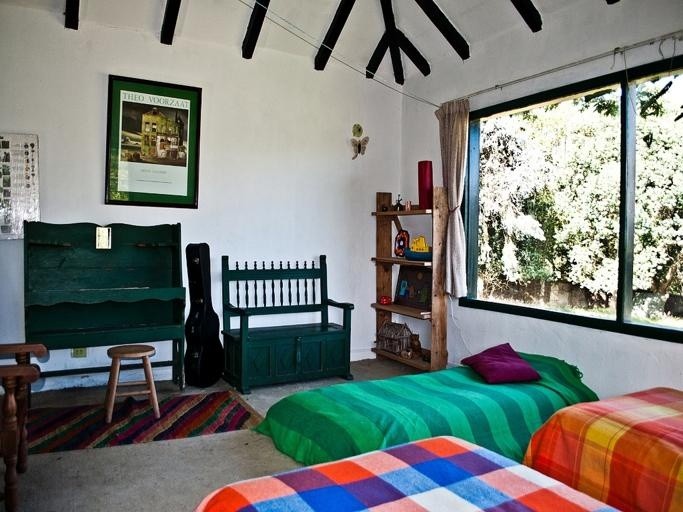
393;200;404;211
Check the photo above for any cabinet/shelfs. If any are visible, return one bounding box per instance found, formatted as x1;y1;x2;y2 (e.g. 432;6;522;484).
372;184;447;372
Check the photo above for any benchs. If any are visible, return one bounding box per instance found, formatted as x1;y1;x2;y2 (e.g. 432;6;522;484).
218;253;354;398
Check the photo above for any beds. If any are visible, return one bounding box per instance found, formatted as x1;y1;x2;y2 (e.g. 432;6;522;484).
522;384;682;508
249;349;602;468
208;433;626;508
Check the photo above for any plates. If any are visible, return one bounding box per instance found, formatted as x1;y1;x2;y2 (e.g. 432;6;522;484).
394;230;410;257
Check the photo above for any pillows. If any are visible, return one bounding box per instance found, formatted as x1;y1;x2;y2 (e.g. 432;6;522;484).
459;342;542;387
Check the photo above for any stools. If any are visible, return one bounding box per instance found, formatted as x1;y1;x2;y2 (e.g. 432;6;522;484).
100;341;163;425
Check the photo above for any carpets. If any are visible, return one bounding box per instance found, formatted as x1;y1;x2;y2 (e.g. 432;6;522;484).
19;386;265;458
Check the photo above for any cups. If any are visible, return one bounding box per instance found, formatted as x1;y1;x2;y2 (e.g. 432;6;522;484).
405;200;411;211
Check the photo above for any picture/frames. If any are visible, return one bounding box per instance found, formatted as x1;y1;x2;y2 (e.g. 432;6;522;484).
102;71;200;210
